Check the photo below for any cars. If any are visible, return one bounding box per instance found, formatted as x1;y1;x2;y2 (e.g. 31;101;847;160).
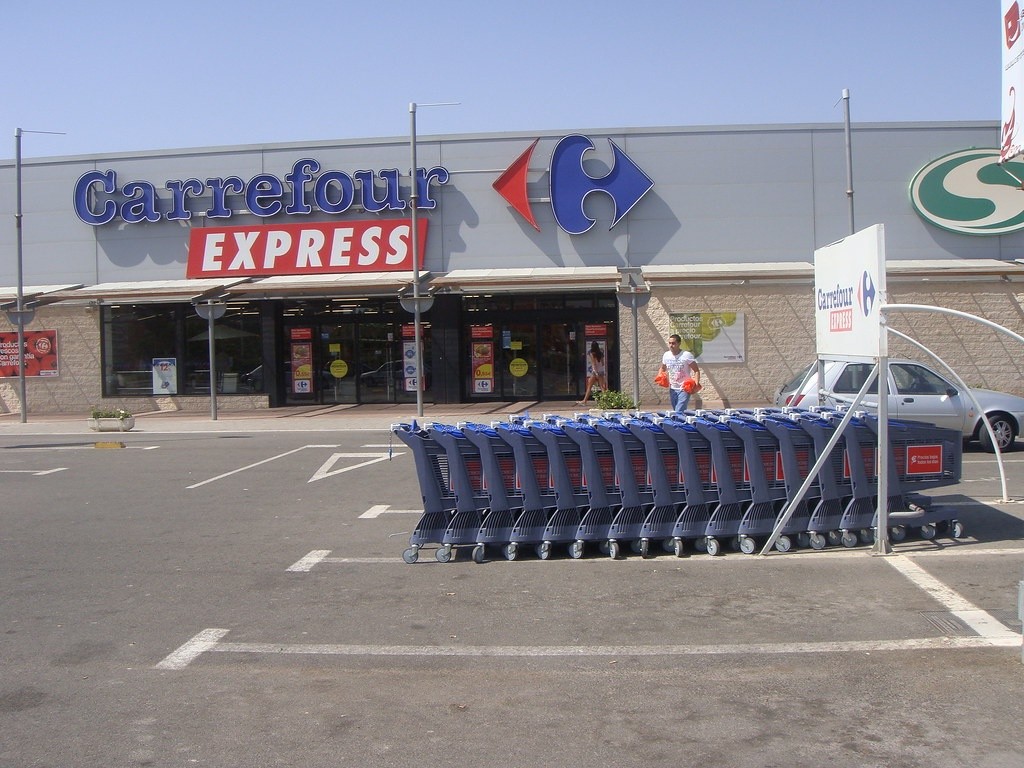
359;359;433;390
771;356;1024;454
239;362;341;394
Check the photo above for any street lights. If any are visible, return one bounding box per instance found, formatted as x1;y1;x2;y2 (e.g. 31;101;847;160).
833;88;857;237
409;101;464;417
13;127;68;424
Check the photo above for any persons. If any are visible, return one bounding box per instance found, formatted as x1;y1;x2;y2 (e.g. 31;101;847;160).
659;334;700;412
576;340;608;407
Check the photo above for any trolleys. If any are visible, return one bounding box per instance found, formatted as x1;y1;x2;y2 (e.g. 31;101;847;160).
392;406;966;568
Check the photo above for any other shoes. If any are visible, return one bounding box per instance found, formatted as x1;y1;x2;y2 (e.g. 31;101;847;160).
575;401;585;407
